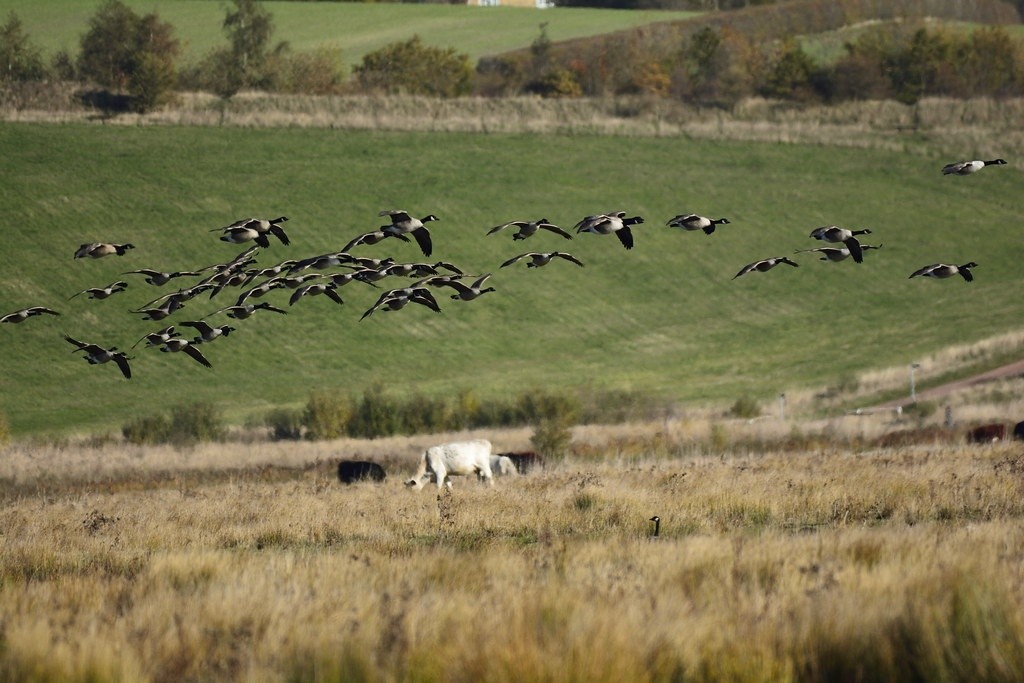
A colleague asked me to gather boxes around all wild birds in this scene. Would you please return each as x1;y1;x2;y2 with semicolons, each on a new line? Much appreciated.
648;515;661;537
730;254;800;280
941;158;1008;176
908;261;979;282
792;225;883;265
0;208;732;382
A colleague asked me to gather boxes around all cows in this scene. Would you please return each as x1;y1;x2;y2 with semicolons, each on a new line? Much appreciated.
402;437;547;492
337;461;388;485
966;420;1024;443
